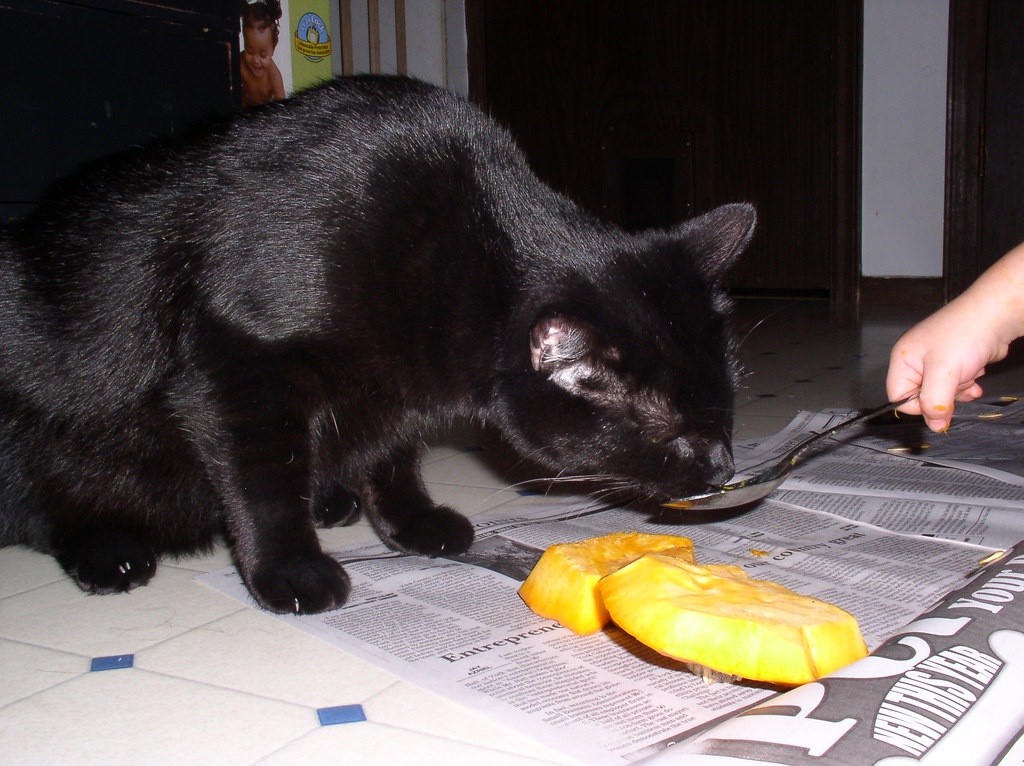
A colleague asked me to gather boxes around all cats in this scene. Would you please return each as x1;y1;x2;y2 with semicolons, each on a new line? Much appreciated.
0;70;758;616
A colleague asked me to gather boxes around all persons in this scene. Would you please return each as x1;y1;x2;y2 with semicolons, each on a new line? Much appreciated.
238;0;285;104
887;241;1024;433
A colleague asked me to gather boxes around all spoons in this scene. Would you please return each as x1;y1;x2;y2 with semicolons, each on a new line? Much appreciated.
658;384;923;511
